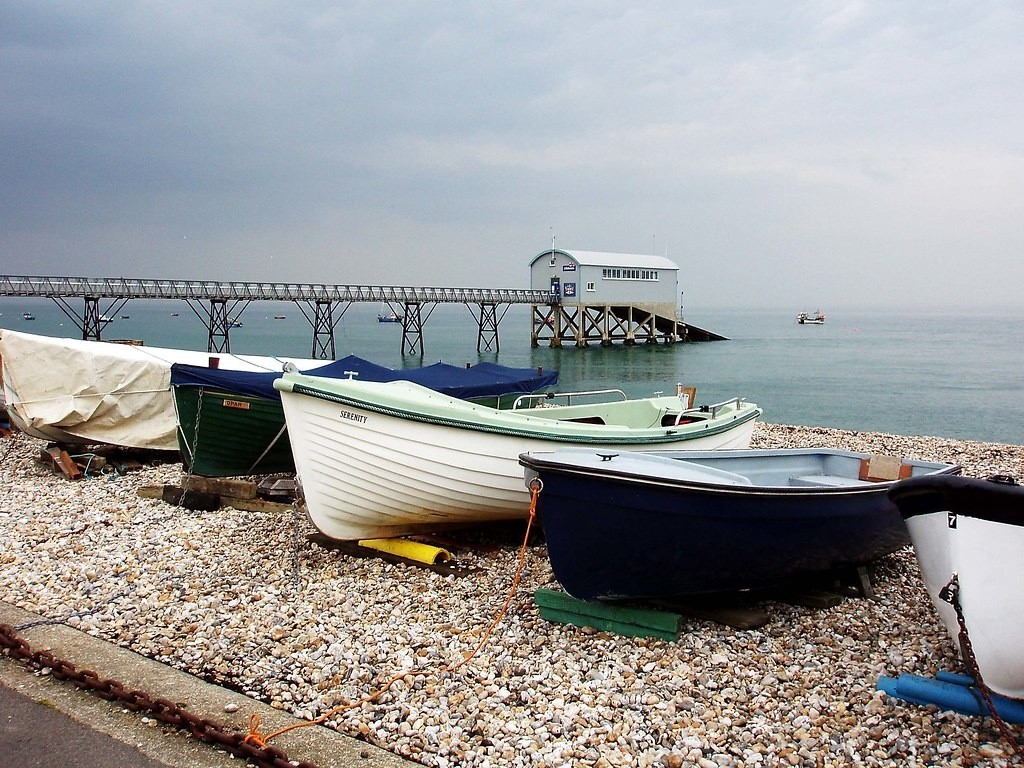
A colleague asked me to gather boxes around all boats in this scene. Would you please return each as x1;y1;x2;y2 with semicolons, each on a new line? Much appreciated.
166;353;562;481
517;444;963;610
884;471;1024;728
0;329;340;445
377;313;404;323
796;312;826;325
271;361;764;544
225;321;244;329
